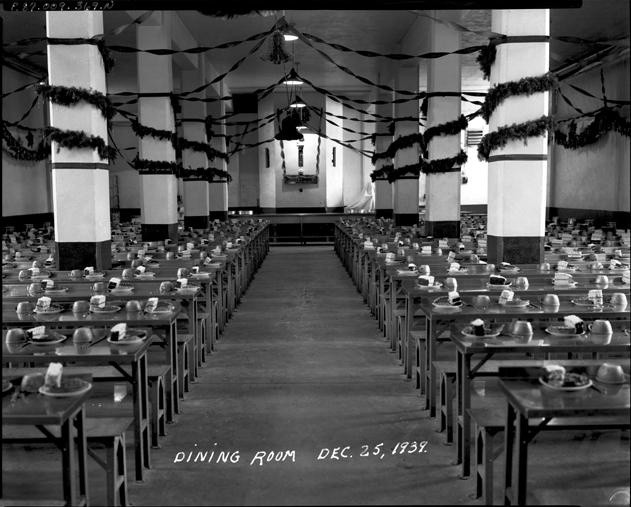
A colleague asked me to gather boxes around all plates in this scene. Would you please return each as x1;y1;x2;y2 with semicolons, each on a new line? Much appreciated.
545;325;586;337
39;381;92;394
462;327;502;338
538;372;593;391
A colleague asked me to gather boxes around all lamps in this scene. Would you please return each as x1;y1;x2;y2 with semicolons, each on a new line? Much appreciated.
261;10;309;141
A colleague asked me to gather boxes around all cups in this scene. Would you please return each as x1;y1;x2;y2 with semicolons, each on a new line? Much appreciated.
3;217;267;346
597;361;627;384
588;320;615;335
512;320;534;337
20;371;44;393
342;212;630;309
